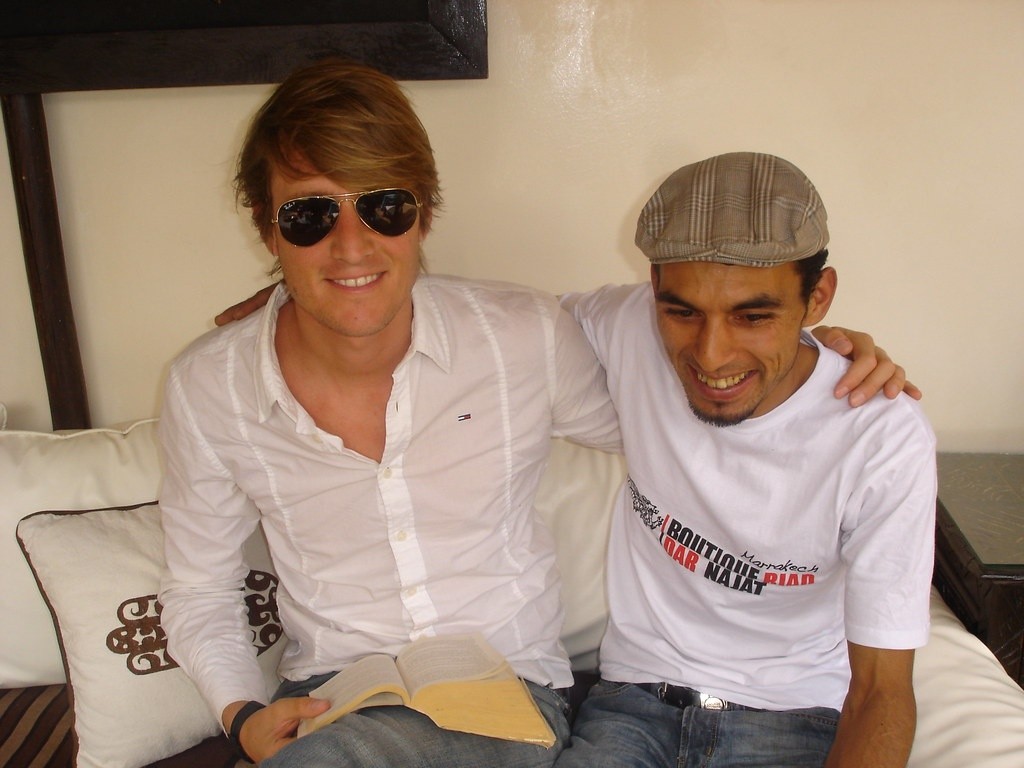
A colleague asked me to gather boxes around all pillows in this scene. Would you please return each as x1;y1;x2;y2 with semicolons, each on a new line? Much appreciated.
16;498;293;768
0;418;159;688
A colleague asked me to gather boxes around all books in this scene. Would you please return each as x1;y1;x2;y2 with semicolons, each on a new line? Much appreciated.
295;632;557;749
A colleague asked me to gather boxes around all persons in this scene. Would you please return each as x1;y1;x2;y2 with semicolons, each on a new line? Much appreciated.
159;60;924;768
211;152;937;768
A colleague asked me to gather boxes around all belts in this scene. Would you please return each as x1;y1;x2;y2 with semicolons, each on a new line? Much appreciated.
639;682;769;714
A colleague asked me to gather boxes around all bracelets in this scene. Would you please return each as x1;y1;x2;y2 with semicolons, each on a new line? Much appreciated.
230;700;265;764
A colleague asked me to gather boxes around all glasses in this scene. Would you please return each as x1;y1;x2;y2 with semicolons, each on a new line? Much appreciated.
271;187;424;247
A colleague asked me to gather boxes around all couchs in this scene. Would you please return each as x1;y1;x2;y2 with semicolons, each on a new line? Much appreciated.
0;592;1024;768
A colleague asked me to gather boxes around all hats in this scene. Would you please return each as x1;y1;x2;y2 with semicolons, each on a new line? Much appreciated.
634;151;830;268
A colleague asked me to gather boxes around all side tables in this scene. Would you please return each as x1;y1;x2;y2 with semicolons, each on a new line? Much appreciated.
937;452;1024;687
